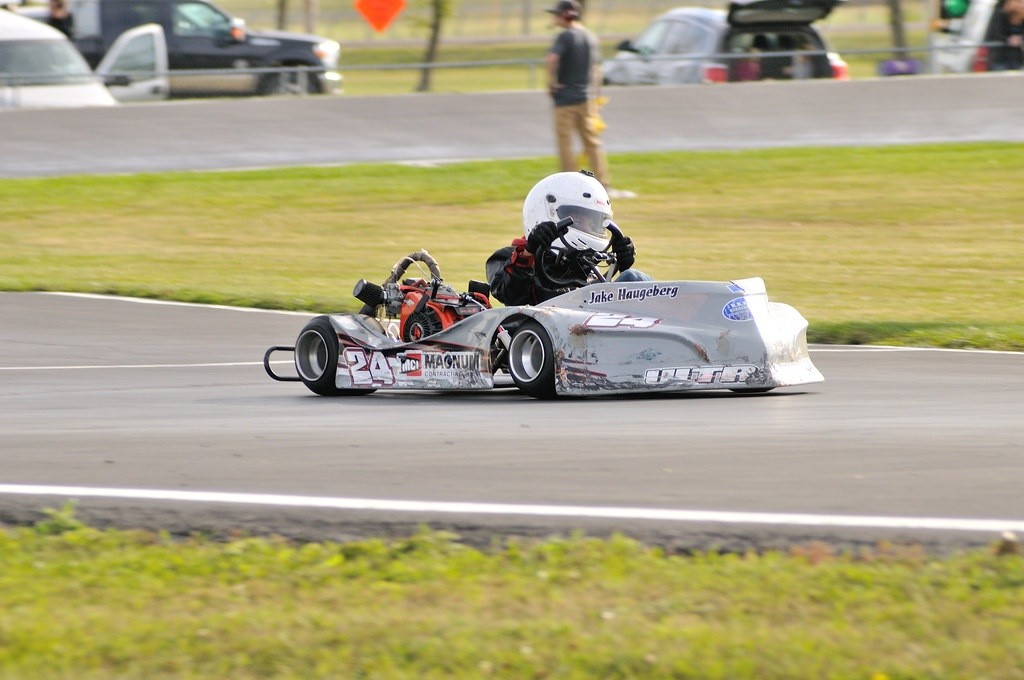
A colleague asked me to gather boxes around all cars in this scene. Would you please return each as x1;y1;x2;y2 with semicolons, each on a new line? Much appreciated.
930;0;1023;78
599;1;852;86
0;3;169;110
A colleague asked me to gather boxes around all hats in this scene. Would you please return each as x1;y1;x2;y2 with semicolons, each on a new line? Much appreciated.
546;1;582;19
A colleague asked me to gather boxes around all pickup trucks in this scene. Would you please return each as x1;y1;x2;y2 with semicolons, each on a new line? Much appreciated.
24;0;343;100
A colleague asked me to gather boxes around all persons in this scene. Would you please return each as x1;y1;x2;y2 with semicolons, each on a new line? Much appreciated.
783;44;819;79
544;1;638;198
485;171;653;306
47;0;73;38
930;0;971;74
732;47;773;82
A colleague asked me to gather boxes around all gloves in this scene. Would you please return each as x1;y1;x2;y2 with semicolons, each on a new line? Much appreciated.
525;221;569;256
611;237;635;272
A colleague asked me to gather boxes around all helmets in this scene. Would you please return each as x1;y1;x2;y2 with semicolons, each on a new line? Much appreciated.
522;169;613;255
